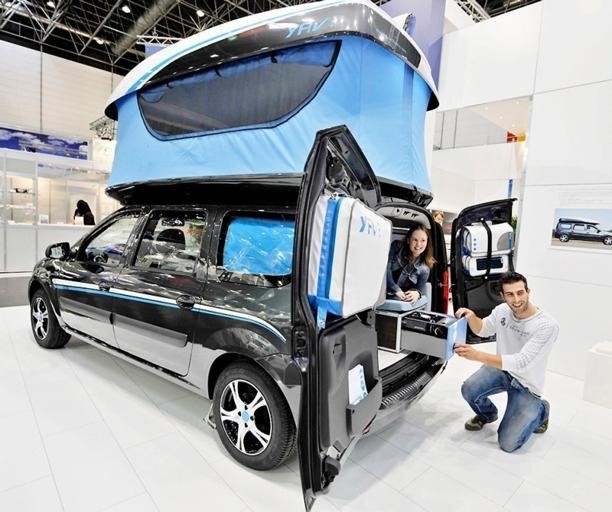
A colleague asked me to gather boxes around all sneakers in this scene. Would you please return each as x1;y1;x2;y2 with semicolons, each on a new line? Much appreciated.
465;414;497;430
534;400;549;433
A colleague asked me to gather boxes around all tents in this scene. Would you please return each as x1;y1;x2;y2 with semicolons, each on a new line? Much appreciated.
103;0;440;209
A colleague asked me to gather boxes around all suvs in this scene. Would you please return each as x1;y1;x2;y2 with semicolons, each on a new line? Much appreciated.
21;123;521;512
554;218;612;246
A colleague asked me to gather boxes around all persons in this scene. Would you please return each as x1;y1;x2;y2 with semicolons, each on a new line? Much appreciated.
73;200;95;225
387;224;438;305
453;272;559;453
434;211;445;224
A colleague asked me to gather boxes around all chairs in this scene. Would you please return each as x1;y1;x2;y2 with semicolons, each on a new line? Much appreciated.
158;230;185;255
